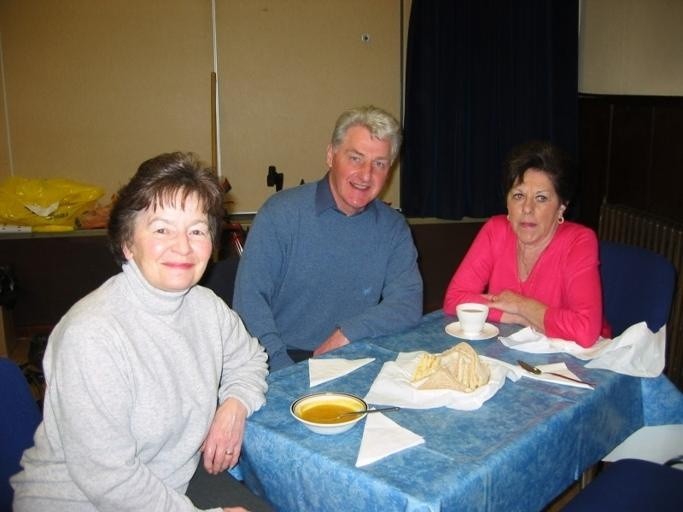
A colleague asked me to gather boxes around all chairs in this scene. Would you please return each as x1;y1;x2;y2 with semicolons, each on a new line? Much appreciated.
0;353;45;511
591;245;675;360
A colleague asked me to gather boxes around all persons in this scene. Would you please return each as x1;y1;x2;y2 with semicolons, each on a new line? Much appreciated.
443;146;612;348
232;105;424;373
9;152;269;512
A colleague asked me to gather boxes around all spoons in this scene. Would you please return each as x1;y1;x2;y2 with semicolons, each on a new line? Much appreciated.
516;358;598;390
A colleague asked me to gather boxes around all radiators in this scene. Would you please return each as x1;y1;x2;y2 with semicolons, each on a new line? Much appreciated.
589;198;681;387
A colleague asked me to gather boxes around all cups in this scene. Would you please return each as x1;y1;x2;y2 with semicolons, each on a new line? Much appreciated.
456;304;489;332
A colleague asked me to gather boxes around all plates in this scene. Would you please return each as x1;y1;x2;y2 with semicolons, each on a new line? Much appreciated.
444;321;500;343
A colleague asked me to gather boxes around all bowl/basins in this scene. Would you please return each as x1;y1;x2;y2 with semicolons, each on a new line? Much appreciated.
289;393;367;437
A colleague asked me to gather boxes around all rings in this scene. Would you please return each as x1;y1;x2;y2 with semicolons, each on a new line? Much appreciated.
492;296;497;301
226;451;233;455
